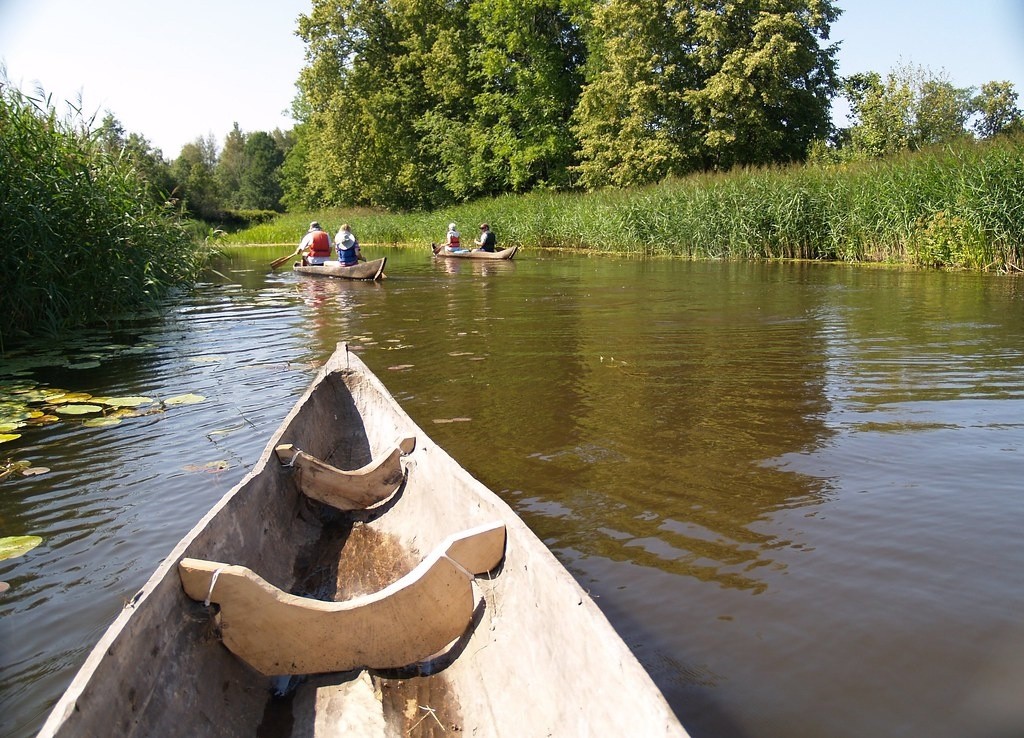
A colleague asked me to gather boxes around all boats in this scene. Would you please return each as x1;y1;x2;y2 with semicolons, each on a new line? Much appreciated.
293;257;387;283
430;240;517;261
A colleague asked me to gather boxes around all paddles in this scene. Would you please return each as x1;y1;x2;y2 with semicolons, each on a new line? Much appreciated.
432;240;448;256
269;246;308;271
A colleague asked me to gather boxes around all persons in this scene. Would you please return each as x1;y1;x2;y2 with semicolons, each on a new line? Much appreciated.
333;224;367;266
440;223;461;254
471;223;497;253
296;222;332;266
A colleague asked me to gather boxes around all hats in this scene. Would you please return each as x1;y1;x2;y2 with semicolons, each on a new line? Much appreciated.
335;230;355;250
308;222;322;233
449;223;456;231
479;224;489;230
